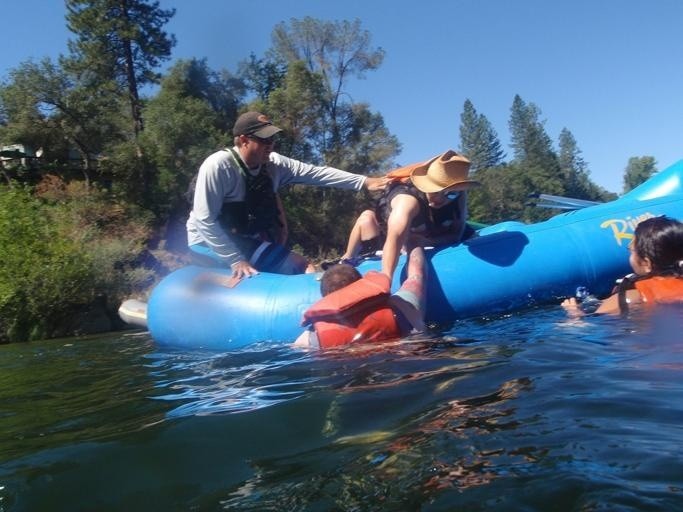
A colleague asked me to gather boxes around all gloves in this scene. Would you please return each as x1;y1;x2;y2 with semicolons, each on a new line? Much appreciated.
140;155;683;352
115;295;148;329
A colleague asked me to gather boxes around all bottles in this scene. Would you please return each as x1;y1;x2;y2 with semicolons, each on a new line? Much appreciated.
411;150;481;193
233;111;282;139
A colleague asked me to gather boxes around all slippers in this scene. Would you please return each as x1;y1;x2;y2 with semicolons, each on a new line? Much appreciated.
229;149;283;242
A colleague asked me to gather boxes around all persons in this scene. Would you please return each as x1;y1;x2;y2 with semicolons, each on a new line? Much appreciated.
183;111;394;277
560;215;683;315
293;242;425;347
319;149;483;271
